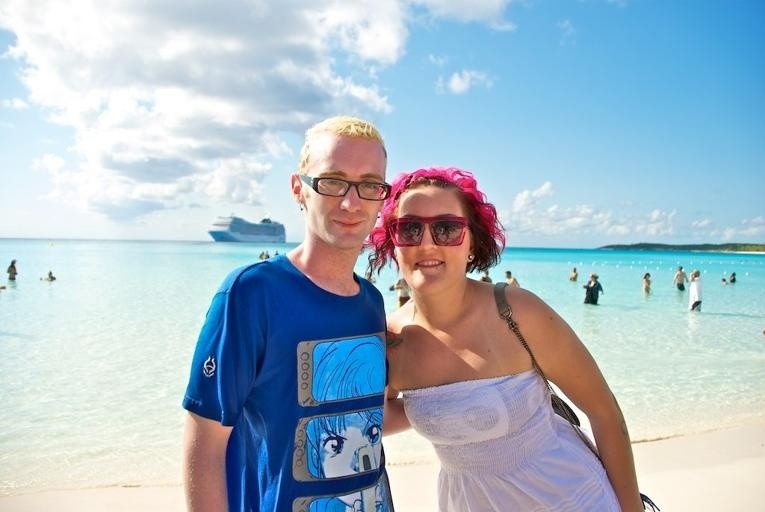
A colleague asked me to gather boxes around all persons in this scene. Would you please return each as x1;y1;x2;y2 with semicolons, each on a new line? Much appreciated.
6;259;18;280
361;167;658;511
389;276;411;306
39;270;56;282
183;117;411;512
480;266;736;311
259;249;279;260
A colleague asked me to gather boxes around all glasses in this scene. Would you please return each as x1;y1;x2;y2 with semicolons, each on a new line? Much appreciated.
386;216;468;246
298;171;392;200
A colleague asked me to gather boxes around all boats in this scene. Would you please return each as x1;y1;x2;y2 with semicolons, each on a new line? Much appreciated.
208;216;285;242
